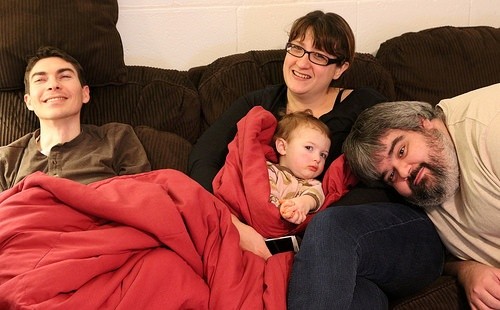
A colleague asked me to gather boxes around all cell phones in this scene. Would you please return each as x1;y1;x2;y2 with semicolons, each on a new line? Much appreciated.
265;235;299;255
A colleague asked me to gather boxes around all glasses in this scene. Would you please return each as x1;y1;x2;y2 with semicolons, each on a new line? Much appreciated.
284;43;345;66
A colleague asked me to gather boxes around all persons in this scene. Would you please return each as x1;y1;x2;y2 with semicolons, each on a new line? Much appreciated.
260;110;332;224
342;77;500;310
0;45;151;200
191;10;444;309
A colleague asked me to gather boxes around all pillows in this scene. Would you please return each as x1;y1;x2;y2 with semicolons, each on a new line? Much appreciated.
0;0;127;93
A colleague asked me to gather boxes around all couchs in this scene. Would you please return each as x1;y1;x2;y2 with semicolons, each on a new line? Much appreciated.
0;23;499;310
